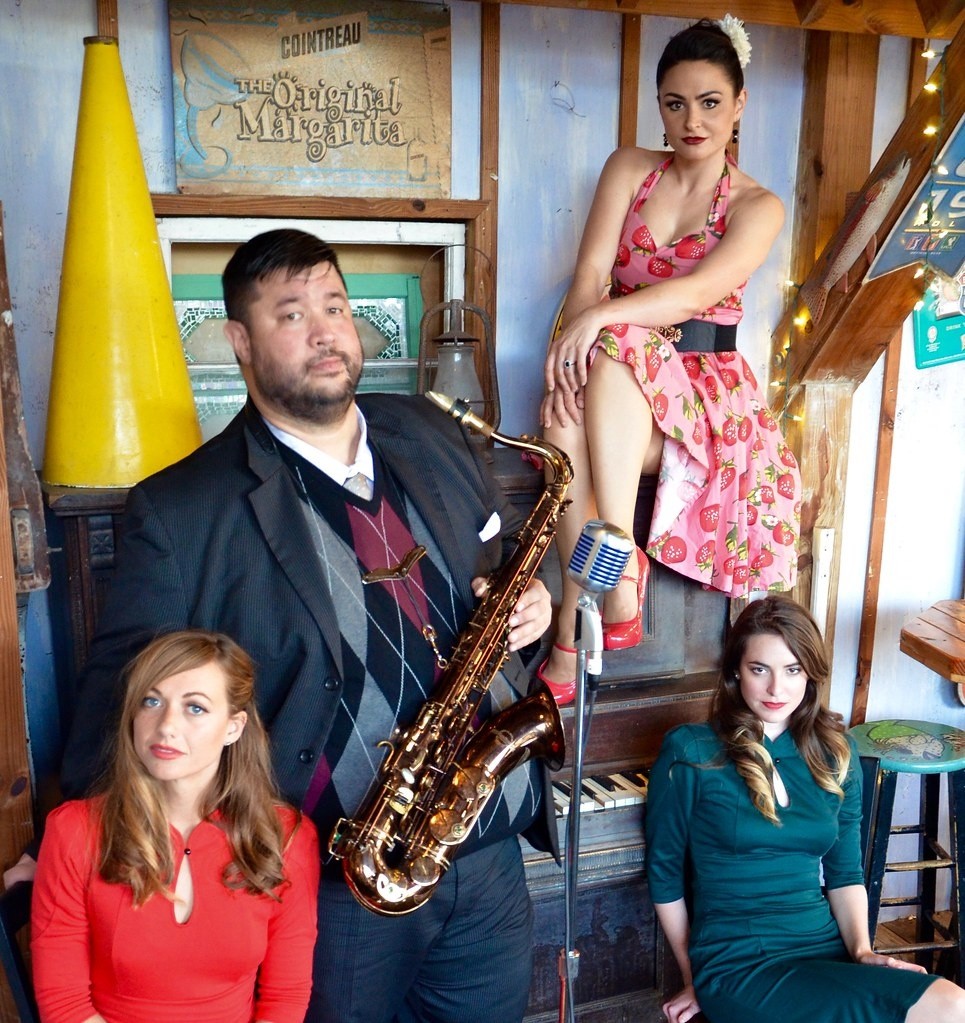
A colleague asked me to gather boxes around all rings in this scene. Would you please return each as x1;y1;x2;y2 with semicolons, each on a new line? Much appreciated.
564;360;576;368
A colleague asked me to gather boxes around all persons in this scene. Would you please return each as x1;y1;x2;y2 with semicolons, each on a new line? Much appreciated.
30;630;320;1023
540;16;801;704
3;230;564;1023
638;595;964;1023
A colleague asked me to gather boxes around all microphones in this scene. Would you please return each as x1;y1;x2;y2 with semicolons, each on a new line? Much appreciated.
567;518;637;690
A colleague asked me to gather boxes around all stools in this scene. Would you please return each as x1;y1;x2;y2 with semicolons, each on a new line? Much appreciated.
847;720;965;957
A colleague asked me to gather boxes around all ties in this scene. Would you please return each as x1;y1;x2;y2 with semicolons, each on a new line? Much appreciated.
345;472;375;501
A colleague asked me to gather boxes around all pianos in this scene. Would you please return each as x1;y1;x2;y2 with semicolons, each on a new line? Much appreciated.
32;444;753;1023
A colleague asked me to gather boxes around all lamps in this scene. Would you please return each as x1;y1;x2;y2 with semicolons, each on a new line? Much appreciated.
416;244;501;465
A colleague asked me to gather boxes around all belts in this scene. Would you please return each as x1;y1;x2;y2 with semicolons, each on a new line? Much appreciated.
669;318;737;351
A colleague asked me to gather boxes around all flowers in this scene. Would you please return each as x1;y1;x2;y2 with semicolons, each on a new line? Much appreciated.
716;14;751;68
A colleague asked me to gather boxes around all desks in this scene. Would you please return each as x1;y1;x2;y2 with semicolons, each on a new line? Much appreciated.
899;601;965;682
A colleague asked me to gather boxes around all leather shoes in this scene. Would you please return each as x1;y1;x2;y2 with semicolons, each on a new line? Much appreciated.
536;642;592;706
600;545;650;651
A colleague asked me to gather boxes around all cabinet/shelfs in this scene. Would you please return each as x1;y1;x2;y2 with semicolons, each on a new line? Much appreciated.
37;470;129;681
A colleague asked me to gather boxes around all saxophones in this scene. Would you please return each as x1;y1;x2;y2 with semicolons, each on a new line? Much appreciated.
325;389;576;918
